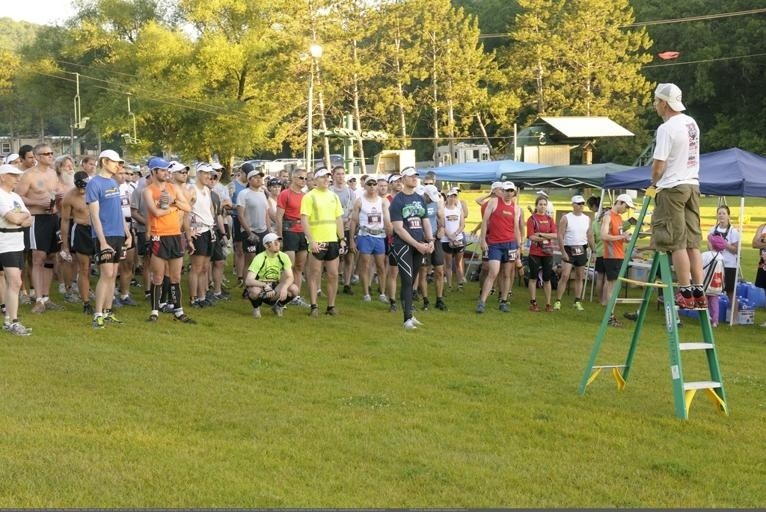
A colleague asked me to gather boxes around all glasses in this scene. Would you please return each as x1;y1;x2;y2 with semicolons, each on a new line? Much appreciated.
180;171;189;176
575;202;587;207
9;159;23;165
505;189;515;194
424;182;435;186
124;172;135;177
37;152;55;157
266;238;282;245
27;155;36;160
282;174;290;179
365;182;379;187
214;169;224;175
208;177;217;182
293;175;309;182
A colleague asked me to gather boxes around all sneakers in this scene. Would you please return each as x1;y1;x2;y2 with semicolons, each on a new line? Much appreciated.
325;307;339;316
662;321;682;329
149;310;159;322
130;280;143;288
198;299;208;308
45;299;66;313
158;300;167;312
88;289;96;300
435;301;448;313
694;296;709;309
545;304;554;313
712;323;718;329
253;307;261;320
0;305;8;315
189;301;201;311
403;318;419;331
105;312;124;326
623;310;638;322
72;282;79;292
119;295;136;307
390;303;398;314
379;295;389;305
343;284;355;296
174;312;196;325
411;289;420;302
607;315;624;328
215;293;228;301
499;302;510;313
553;301;561;311
657;293;696;310
63;291;82;305
310;308;319;321
475;301;487;314
222;276;232;283
423;303;430;312
31;300;47;315
92;312;107;330
161;304;175;314
274;304;285;318
58;281;67;295
364;293;372;303
2;320;34;337
221;290;233;300
292;297;311;309
573;301;585;312
83;302;91;316
221;282;230;289
317;289;327;299
373;275;379;285
529;302;540;313
112;298;124;308
350;273;360;284
115;287;122;292
19;293;31;307
31;292;39;302
411;316;424;327
205;291;220;305
144;293;152;301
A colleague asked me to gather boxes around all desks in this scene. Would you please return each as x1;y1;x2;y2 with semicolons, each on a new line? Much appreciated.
625;261;664;312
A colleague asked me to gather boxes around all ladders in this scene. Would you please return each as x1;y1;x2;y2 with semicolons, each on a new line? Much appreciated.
580;185;731;419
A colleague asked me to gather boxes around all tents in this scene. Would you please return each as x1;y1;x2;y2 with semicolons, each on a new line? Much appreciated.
415;159;551;183
604;146;766;328
501;161;650;301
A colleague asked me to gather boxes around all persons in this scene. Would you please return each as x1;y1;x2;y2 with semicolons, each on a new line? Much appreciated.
526;196;557;313
141;157;192;324
236;170;270;299
131;165;142;183
450;186;468;219
276;168;308;296
86;149;132;330
3;153;23;168
552;195;596;313
427;172;437;184
475;181;521;313
267;177;283;236
54;154;96;303
82;156;96;177
208;168;228;309
389;166;424;330
442;190;466;293
375;178;389;293
528;190;554;218
210;162;233;304
304;172;315;190
15;145;37;307
300;166;345;318
422;175;434;186
355;175;369;196
476;182;502;303
184;161;214;310
230;168;240;178
61;170;95;316
649;83;708;310
419;186;449;312
130;174;170;302
349;177;393;305
708;205;738;300
586;195;601;218
751;223;766;290
591;206;611;307
125;166;136;191
600;194;636;328
0;163;33;337
245;232;298;318
113;164;137;306
17;144;66;315
497;207;525;307
166;163;196;313
701;234;728;329
278;170;290;189
329;166;355;296
227;163;256;287
386;172;418;313
347;175;360;197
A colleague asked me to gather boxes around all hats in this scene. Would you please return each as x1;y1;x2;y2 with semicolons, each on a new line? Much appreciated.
401;167;421;178
500;181;518;192
211;161;226;170
364;176;378;184
207;164;215;173
196;162;215;173
571;195;586;205
376;175;389;183
488;182;502;197
415;185;425;196
707;233;727;252
536;190;550;198
268;177;283;187
73;171;90;189
447;191;459;197
149;158;172;174
99;149;126;166
169;164;191;174
450;187;462;193
616;193;636;209
247;170;265;183
423;184;441;204
346;175;358;186
388;174;402;185
6;153;19;164
169;160;181;165
654;82;687;112
313;168;333;179
2;156;7;164
0;163;25;176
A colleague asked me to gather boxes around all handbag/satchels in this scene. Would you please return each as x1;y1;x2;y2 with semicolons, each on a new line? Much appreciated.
359;225;385;236
0;227;31;252
566;244;586;256
448;233;463;248
282;220;302;232
531;212;554;250
